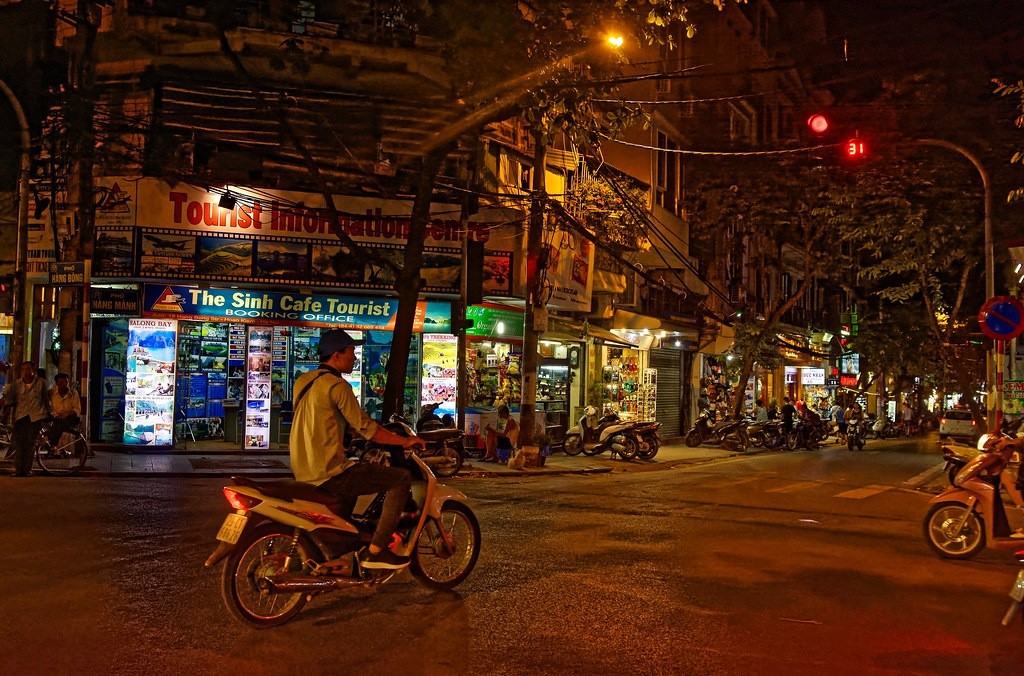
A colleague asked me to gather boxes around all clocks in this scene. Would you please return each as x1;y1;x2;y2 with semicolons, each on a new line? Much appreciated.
569;346;579;369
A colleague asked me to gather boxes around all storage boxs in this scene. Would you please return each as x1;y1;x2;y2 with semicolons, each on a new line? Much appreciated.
515;446;539;466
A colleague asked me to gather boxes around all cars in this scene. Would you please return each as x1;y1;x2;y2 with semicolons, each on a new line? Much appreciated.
939;407;987;441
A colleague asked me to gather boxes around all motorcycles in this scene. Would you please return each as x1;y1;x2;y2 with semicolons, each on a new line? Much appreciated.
562;404;664;462
922;417;1024;625
685;404;830;451
204;423;482;630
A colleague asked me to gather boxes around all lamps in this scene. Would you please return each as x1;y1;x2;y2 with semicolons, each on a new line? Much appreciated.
217;182;237;210
490;341;496;348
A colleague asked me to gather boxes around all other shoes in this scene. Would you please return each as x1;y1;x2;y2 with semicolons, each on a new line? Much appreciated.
15;468;32;477
478;455;498;463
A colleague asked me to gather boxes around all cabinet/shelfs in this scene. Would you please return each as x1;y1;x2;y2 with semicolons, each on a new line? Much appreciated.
535;364;567;404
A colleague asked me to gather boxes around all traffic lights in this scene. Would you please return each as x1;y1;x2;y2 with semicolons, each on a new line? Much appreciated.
805;114;833;137
0;283;13;294
843;139;868;160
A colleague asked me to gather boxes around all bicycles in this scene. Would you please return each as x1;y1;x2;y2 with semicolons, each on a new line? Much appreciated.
0;418;89;477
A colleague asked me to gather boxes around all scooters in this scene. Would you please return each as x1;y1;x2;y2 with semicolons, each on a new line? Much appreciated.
848;418;865;451
862;415;893;440
359;401;464;479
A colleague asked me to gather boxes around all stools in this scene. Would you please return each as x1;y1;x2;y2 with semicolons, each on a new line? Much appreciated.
540;442;552;457
497;447;515;465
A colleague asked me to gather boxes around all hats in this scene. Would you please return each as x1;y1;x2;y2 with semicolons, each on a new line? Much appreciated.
316;330;365;353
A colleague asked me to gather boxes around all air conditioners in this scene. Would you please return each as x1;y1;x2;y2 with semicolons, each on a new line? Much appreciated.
577;295;614;318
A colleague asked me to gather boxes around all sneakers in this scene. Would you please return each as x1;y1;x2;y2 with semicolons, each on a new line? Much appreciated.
361;547;411;570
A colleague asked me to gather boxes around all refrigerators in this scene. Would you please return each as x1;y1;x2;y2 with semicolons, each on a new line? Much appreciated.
464;406;546;442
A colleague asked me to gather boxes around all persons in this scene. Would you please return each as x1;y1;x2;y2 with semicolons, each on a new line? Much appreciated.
829;400;866;445
3;362;48;478
781;396;823;447
844;357;857;374
289;329;426;570
901;401;916;437
42;373;81;452
478;405;519;463
750;399;780;421
996;435;1024;540
271;378;285;406
